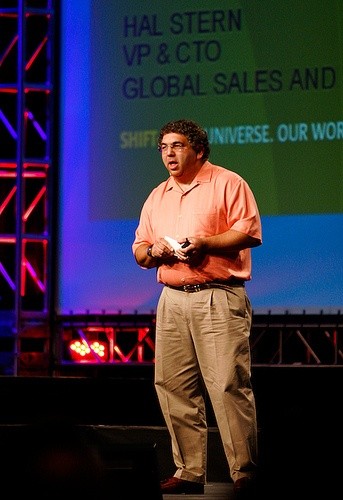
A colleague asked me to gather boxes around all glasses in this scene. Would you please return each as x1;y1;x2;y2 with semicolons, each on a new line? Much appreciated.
160;144;191;153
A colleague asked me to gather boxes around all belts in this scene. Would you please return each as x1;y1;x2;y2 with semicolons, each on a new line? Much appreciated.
164;281;242;293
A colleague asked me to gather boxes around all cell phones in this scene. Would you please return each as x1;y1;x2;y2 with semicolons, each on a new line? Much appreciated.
182;238;190;254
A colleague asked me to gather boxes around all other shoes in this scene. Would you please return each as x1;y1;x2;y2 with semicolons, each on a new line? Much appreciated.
234;477;250;494
160;477;204;495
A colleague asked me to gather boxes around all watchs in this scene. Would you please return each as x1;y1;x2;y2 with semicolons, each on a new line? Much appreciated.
146;243;155;258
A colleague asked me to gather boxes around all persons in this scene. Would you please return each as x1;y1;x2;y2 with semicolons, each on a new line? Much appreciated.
131;117;264;494
